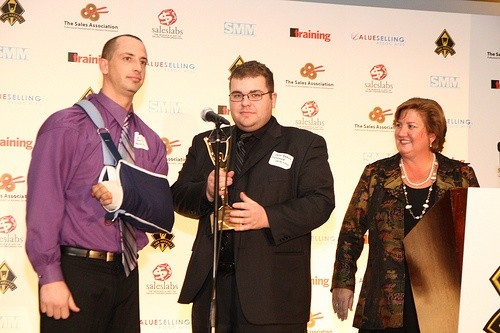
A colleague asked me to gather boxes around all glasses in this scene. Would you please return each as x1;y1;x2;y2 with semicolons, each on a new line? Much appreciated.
228;91;273;102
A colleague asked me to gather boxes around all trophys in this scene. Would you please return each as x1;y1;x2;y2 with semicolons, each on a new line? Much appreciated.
203;126;242;233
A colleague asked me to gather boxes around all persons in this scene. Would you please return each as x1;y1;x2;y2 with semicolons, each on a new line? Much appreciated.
330;98;480;333
24;35;168;333
170;59;336;333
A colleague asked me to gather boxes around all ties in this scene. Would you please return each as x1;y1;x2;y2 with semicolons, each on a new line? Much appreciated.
220;131;255;252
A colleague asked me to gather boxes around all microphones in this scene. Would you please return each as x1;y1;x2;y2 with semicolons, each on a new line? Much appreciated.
200;107;230;126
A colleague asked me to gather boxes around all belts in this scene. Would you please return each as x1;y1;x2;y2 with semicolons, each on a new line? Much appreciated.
217;261;236;273
60;244;139;263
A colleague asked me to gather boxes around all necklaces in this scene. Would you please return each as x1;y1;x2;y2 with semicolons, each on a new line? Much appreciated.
401;153;436;186
399;160;438;219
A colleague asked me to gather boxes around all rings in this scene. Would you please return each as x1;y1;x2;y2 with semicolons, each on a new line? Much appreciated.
334;304;339;306
